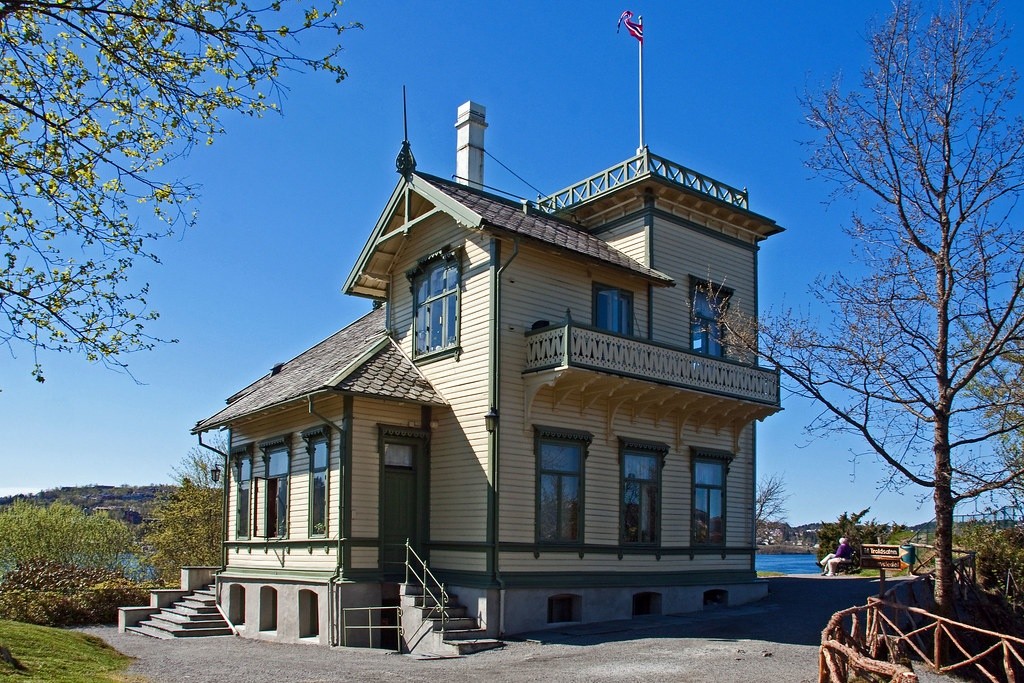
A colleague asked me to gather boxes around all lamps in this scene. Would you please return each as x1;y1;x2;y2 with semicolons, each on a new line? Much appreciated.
484;403;500;435
211;464;224;483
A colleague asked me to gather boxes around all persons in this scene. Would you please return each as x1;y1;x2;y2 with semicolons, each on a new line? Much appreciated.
815;537;852;576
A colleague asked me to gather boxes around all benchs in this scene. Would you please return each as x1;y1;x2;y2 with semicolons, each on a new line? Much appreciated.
835;550;856;574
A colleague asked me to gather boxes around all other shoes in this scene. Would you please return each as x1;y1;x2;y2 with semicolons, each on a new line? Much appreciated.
816;561;823;569
826;573;834;577
821;572;828;575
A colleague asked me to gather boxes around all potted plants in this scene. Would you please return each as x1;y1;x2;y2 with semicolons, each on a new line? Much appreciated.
314;523;325;534
274;525;284;537
